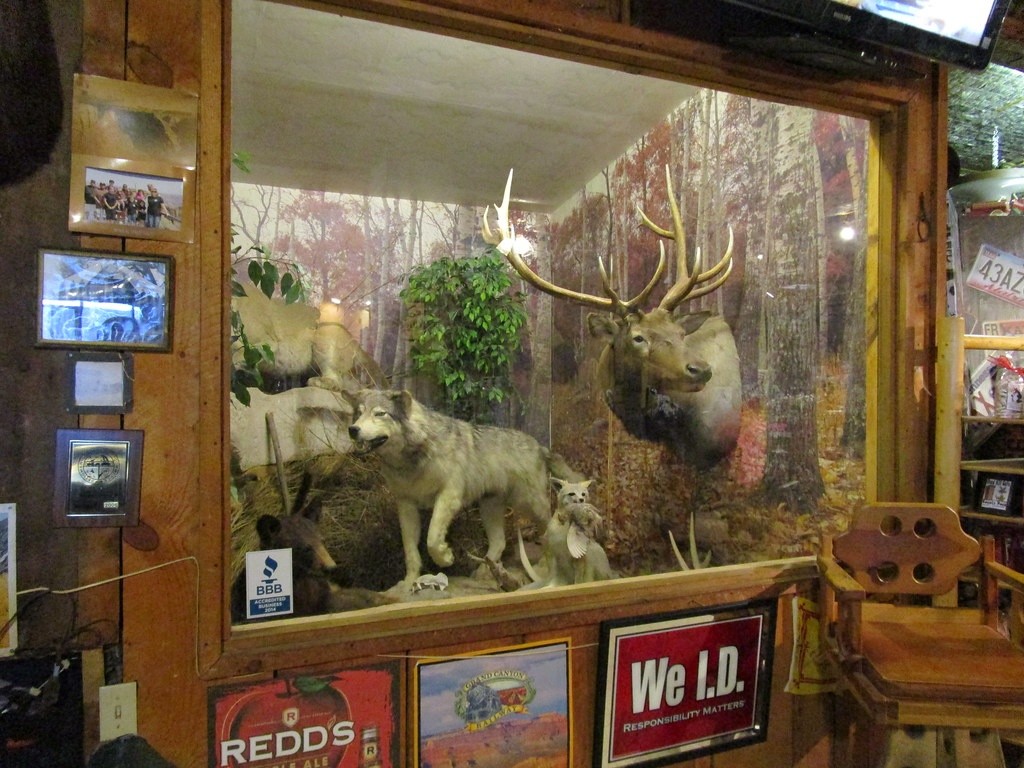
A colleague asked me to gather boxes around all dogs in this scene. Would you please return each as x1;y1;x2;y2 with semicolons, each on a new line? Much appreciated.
340;387;587;591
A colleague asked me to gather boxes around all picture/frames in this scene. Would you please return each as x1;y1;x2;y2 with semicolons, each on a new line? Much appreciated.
590;595;781;767
34;246;177;354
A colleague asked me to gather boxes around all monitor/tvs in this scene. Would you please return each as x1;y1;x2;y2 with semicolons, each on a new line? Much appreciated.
723;0;1012;73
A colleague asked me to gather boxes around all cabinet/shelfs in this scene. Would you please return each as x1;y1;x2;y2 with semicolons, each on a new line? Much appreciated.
932;314;1022;609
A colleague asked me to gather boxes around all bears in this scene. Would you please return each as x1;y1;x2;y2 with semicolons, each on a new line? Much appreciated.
230;495;338;629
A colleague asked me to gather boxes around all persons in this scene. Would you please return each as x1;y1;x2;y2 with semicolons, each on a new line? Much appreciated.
84;180;170;227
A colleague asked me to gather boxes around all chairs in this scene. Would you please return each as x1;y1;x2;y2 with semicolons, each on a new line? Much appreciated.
814;500;1024;767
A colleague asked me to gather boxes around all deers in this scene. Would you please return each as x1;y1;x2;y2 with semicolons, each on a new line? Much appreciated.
481;161;744;468
